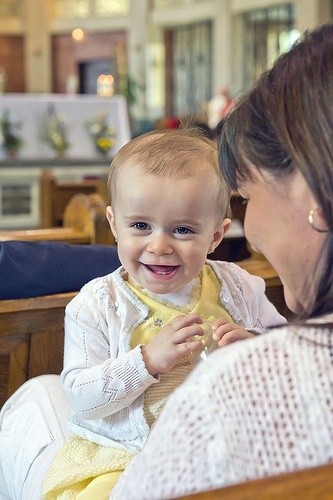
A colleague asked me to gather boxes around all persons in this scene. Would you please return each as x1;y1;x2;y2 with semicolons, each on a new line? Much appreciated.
0;22;333;500
60;129;288;452
206;85;232;130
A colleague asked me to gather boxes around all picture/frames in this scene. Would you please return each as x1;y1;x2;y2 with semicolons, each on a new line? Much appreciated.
0;94;130;167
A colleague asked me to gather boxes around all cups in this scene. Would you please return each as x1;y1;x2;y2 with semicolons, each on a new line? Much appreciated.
186;320;223;368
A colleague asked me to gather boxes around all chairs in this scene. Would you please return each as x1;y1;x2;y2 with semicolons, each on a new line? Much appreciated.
1;170;332;500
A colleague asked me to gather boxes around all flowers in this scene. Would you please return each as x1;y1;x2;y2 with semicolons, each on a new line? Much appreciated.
37;111;69;153
0;110;25;153
86;109;116;152
